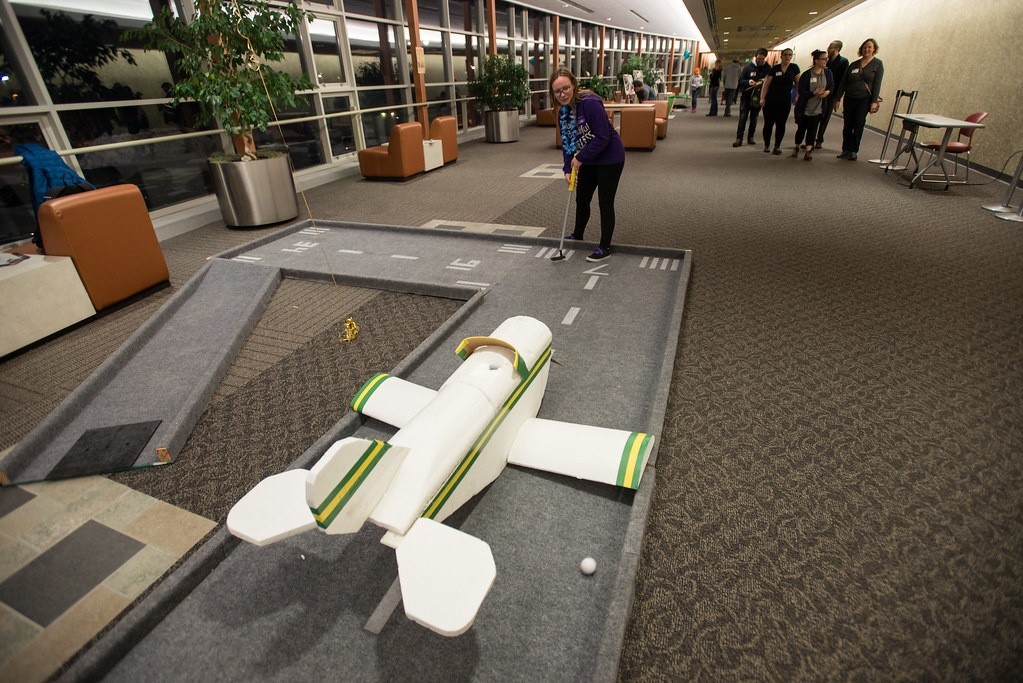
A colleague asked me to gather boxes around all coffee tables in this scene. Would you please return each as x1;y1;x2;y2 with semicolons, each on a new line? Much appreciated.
381;140;444;171
0;252;97;359
603;103;655;136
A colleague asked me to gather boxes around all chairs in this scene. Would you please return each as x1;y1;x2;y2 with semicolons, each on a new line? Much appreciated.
913;113;989;183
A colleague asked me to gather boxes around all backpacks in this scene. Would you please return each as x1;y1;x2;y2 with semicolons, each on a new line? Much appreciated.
28;171;97;251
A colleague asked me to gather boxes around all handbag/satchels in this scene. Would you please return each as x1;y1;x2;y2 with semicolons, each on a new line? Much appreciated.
869;96;885;114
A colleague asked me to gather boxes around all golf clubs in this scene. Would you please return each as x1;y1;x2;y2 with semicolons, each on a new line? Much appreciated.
551;153;578;261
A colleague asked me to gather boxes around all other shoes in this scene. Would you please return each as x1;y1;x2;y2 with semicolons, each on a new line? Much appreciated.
791;147;799;158
732;139;743;147
804;152;812;160
691;108;697;113
813;144;822;149
723;113;732;117
763;147;770;152
836;151;848;158
848;152;858;159
772;149;781;155
800;144;807;149
747;138;755;144
705;113;717;117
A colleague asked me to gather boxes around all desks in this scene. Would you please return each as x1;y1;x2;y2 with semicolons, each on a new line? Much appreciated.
894;113;985;190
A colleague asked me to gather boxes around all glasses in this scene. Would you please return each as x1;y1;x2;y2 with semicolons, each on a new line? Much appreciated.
553;83;573;98
817;58;830;61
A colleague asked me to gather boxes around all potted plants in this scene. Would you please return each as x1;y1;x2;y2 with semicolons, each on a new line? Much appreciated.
120;1;321;227
466;53;533;142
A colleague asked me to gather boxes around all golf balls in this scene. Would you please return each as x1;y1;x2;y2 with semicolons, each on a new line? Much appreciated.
580;558;597;574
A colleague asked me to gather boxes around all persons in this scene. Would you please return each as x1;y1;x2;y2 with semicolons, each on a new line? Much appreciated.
705;60;722;116
5;91;38;150
833;39;884;159
733;48;771;147
791;49;835;161
760;48;800;155
549;68;625;262
800;41;849;150
690;68;704;113
161;83;194;153
95;82;156;160
721;59;743;117
632;81;656;104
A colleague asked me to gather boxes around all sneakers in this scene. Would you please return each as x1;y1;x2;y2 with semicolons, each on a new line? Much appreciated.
586;246;612;263
563;235;584;242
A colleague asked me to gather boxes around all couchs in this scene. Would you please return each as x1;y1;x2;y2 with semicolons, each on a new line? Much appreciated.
536;100;669;152
4;184;169;358
358;115;458;179
